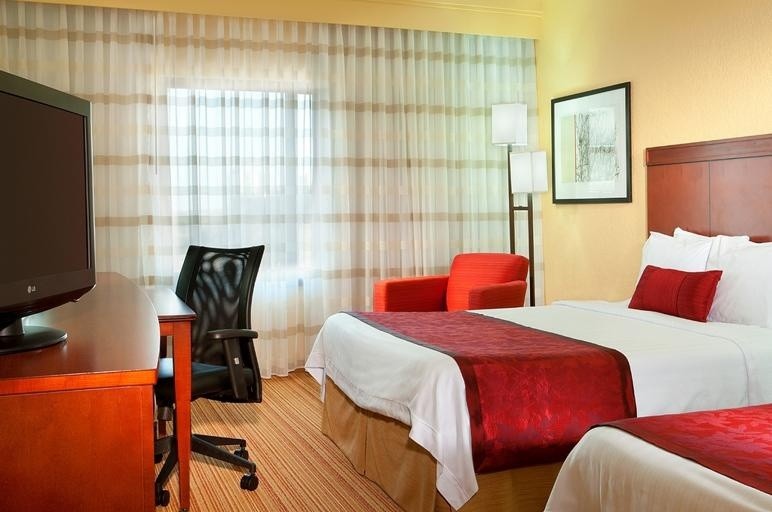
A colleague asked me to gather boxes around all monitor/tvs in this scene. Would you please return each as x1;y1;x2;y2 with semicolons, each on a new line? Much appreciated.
0;69;96;355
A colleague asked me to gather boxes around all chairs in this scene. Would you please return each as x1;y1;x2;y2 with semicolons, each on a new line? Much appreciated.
148;244;270;506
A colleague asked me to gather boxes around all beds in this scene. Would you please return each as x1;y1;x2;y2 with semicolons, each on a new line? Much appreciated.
298;131;772;511
539;401;772;511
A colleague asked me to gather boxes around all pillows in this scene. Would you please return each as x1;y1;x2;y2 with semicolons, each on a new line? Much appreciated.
634;231;714;293
702;232;771;330
673;224;749;247
622;264;722;324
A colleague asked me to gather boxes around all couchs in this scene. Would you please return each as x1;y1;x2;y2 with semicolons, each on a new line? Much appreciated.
371;249;530;313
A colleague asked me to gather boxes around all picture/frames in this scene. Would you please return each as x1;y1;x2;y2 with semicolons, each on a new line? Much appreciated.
545;81;633;205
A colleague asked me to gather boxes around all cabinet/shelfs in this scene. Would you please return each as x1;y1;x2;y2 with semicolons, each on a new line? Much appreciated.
0;270;164;512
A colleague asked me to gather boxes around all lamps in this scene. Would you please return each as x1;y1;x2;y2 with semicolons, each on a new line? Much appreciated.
507;148;550;306
488;101;528;254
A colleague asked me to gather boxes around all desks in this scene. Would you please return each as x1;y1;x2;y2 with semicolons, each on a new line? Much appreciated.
134;283;199;511
